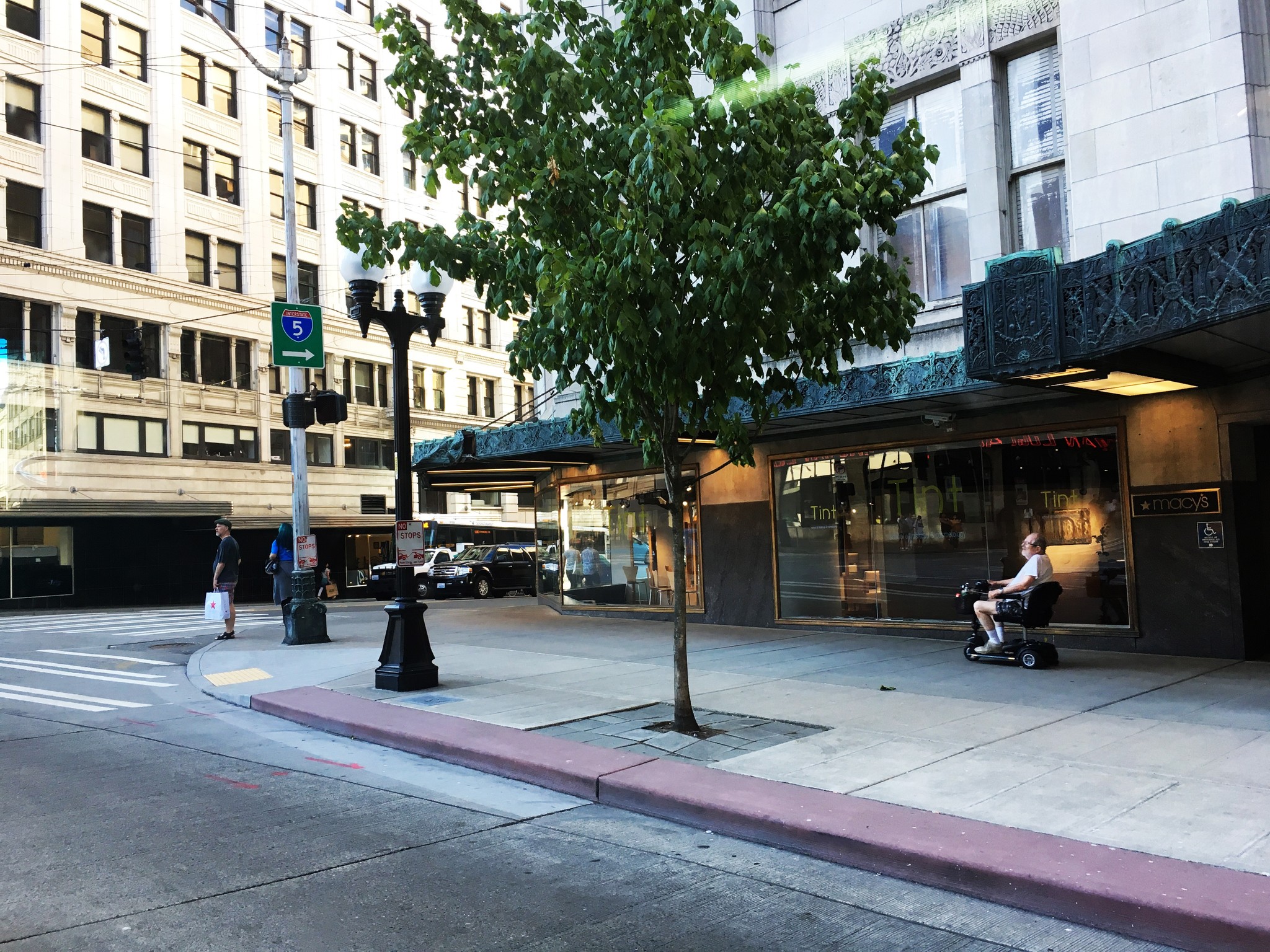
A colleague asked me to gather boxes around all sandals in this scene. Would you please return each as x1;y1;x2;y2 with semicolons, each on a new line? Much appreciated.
214;630;235;640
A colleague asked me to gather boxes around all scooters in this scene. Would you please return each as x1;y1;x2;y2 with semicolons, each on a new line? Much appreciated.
954;577;1063;670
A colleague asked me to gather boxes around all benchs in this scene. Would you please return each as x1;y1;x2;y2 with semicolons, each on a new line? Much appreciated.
845;569;880;611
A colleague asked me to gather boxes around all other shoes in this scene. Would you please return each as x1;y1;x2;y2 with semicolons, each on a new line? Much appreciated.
317;597;321;600
332;596;336;600
974;640;1003;654
326;581;338;597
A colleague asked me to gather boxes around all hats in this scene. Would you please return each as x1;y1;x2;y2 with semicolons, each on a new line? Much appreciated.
214;518;232;529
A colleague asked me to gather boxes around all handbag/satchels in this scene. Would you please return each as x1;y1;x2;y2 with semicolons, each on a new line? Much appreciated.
205;587;230;620
265;544;281;574
572;560;583;576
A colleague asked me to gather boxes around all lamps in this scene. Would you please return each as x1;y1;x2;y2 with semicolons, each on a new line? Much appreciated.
606;500;612;507
573;500;578;505
619;499;625;509
588;499;595;508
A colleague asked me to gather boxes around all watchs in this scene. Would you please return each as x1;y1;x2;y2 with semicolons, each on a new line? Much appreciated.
999;587;1004;594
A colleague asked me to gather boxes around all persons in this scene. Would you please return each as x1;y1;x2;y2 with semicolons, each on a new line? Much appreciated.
897;513;962;551
317;563;336;600
974;533;1053;654
213;519;241;640
563;538;601;588
270;523;294;625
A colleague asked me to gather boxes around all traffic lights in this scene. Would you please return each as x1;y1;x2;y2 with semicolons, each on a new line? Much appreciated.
315;392;348;425
123;337;147;381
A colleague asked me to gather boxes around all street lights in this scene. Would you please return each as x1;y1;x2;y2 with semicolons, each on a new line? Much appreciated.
339;233;457;693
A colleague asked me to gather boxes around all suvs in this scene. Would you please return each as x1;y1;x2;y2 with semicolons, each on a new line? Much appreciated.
368;541;474;601
426;540;558;600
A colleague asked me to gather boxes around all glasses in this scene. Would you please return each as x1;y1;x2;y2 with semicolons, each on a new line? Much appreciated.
1022;540;1042;549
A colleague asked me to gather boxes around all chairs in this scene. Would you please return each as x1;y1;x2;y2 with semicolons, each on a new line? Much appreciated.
666;571;699;607
621;565;649;605
652;569;672;606
646;567;671;605
